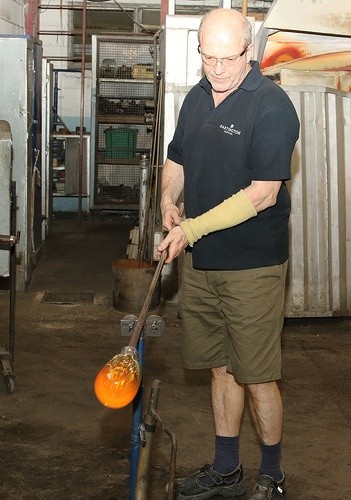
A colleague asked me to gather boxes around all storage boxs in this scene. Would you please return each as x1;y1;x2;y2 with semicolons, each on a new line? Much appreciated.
102;127;139;158
132;63;155;80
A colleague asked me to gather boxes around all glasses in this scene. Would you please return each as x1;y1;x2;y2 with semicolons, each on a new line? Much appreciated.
196;45;248;66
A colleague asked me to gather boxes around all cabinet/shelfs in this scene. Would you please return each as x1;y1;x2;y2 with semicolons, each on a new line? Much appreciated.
88;34;159;223
49;131;88;196
1;33;47;293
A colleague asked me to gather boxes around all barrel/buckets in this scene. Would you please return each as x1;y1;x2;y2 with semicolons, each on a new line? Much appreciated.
111;258;160;314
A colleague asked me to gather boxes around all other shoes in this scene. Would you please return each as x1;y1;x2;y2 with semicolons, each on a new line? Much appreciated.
248;471;286;500
165;463;245;500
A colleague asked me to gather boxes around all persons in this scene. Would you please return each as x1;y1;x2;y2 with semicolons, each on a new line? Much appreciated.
155;8;301;500
